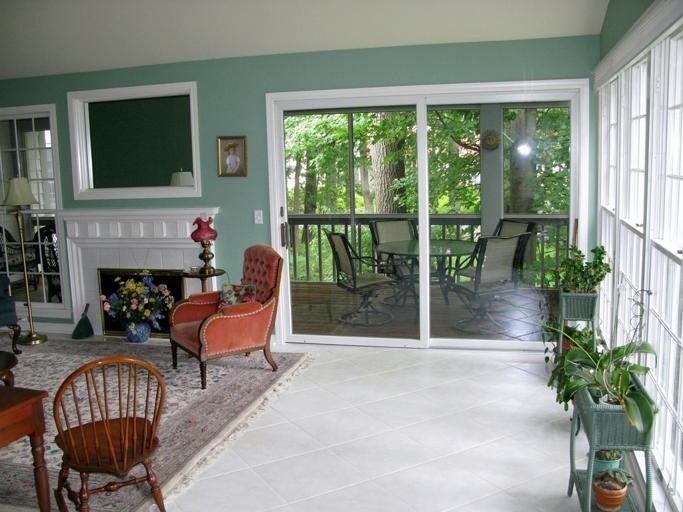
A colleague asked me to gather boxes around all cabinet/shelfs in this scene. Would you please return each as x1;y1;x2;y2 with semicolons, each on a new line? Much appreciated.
568;359;655;512
559;283;598;359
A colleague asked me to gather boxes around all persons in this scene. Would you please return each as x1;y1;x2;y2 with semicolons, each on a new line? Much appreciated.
224;142;241;176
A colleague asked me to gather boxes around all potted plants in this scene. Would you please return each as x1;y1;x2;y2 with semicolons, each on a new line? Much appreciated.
563;324;579;351
599;344;658;434
587;449;623;469
593;469;632;510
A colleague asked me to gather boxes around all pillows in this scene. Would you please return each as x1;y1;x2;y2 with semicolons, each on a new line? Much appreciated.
218;284;256;312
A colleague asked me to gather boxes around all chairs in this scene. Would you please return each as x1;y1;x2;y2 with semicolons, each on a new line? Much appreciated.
169;245;283;389
0;225;40;291
323;217;536;335
0;272;22;355
54;356;165;512
34;226;61;302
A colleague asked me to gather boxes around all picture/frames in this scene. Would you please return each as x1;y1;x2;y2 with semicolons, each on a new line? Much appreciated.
217;136;248;177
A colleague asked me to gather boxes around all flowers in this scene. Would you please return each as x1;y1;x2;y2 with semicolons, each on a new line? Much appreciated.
99;270;174;335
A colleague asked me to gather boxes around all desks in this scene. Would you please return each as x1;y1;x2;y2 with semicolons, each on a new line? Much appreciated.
0;385;51;511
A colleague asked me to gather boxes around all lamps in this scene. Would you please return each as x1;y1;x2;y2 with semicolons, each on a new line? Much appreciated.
1;177;48;346
170;167;194;186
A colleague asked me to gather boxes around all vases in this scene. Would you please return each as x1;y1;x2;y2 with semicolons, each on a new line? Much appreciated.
125;320;151;343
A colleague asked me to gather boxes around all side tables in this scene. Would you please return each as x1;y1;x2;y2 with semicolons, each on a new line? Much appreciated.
181;269;225;293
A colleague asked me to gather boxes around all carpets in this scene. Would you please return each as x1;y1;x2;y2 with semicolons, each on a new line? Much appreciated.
0;332;312;512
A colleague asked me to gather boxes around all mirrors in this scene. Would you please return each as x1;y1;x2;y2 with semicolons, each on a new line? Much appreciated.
66;80;202;201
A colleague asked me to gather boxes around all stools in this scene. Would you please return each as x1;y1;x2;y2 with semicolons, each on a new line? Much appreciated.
0;351;18;387
